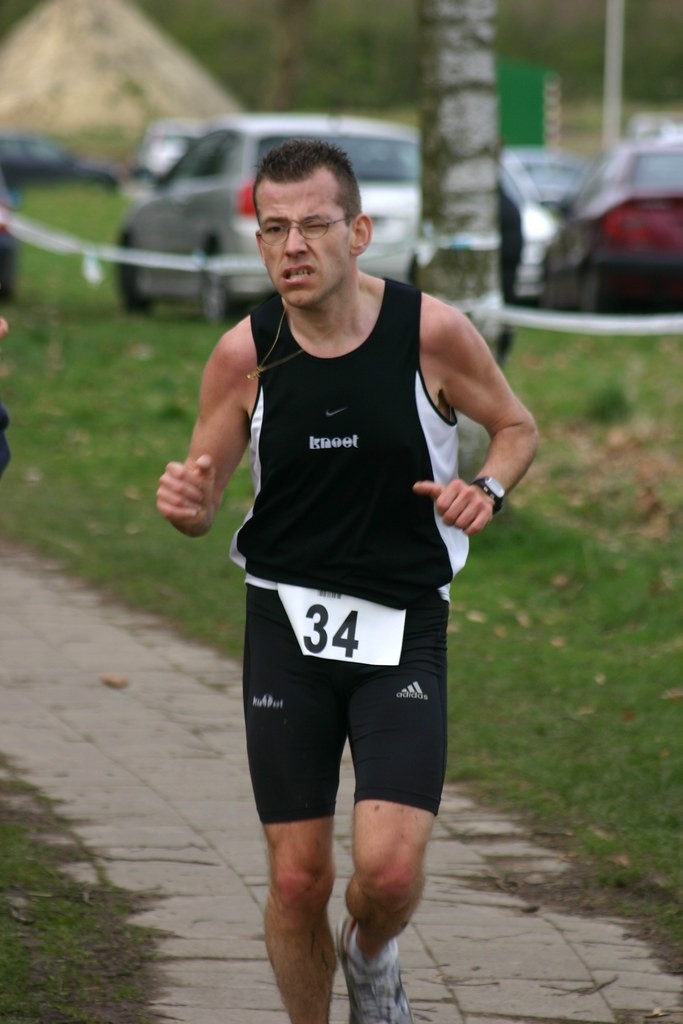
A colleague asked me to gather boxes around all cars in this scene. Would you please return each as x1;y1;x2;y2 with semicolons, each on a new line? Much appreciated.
115;112;422;329
540;126;683;316
0;118;209;321
501;141;596;295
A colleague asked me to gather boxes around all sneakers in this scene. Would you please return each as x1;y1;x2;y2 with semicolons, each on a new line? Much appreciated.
336;913;414;1024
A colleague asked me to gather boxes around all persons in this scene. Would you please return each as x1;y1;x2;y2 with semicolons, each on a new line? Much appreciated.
0;228;15;476
157;138;539;1024
498;137;524;364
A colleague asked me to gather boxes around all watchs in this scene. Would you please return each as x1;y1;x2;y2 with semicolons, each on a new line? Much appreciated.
470;475;505;514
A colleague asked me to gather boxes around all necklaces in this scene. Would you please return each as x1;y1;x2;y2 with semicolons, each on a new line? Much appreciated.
246;276;365;381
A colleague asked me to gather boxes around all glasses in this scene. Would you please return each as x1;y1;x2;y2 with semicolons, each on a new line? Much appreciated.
257;216;358;247
